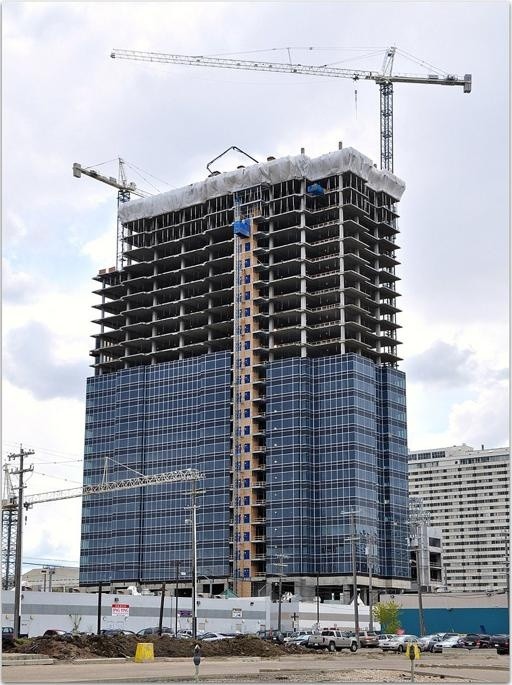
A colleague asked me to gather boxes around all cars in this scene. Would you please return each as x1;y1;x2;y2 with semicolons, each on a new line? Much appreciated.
43;627;237;640
256;628;509;655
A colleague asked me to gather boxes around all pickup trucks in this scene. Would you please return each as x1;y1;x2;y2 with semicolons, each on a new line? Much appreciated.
2;627;28;640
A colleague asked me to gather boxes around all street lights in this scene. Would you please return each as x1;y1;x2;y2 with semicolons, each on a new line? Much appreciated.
184;518;197;639
409;559;425;637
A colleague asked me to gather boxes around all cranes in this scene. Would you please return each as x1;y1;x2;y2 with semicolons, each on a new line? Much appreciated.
1;456;206;590
110;45;473;174
72;156;178;270
